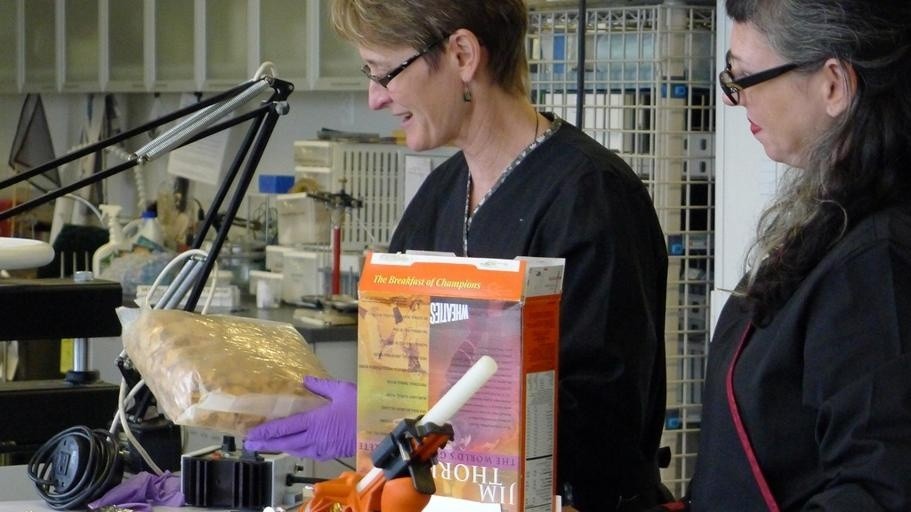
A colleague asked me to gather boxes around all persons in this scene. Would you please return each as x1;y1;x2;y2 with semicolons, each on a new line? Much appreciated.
676;1;910;511
242;1;675;511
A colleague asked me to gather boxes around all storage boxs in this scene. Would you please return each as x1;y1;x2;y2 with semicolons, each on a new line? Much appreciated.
356;248;567;512
264;138;407;309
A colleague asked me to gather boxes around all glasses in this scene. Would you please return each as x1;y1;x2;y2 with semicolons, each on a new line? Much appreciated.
719;50;806;105
360;38;443;88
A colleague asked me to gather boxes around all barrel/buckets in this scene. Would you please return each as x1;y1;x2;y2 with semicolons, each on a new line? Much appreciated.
123;210;166;255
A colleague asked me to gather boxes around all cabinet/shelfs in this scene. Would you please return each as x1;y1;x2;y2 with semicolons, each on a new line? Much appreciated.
524;0;717;500
0;0;369;94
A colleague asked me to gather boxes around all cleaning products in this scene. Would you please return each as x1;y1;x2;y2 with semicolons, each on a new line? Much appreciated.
90;203;132;279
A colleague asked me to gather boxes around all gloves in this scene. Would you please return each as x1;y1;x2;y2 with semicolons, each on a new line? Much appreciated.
87;469;185;512
244;376;357;463
